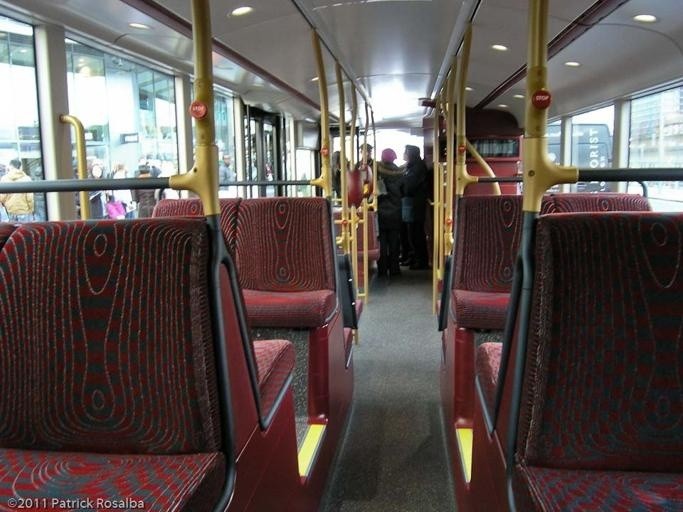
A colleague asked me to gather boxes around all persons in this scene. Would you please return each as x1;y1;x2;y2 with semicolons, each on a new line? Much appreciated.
0;152;166;230
397;145;429;270
332;151;353;207
394;161;412;269
356;143;376;168
372;147;406;282
218;154;235;191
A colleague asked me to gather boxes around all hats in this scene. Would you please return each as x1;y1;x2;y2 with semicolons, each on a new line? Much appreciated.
360;144;372;150
382;149;396;161
407;145;420;158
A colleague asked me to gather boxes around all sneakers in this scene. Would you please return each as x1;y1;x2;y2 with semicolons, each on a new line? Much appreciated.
378;254;429;277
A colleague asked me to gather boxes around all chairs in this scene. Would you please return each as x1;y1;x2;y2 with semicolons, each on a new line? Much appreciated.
0;193;683;512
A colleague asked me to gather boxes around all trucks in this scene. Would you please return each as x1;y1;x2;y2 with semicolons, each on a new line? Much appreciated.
13;116;142;180
542;121;614;195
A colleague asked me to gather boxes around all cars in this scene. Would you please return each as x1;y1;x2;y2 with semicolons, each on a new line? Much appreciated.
216;161;233;190
